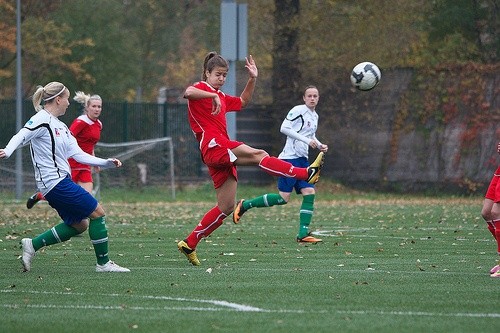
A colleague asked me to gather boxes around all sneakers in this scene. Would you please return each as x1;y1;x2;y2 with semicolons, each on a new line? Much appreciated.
307;152;325;184
27;193;40;209
296;232;322;242
232;199;247;224
490;265;500;273
177;240;200;266
95;260;130;272
20;238;35;271
490;270;500;278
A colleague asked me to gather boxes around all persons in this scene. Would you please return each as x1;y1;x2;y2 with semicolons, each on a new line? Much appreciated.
26;90;102;237
177;51;324;266
481;142;500;277
233;85;328;243
138;158;149;184
0;82;130;272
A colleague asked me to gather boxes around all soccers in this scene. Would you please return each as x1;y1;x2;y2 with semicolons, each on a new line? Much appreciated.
351;61;382;92
